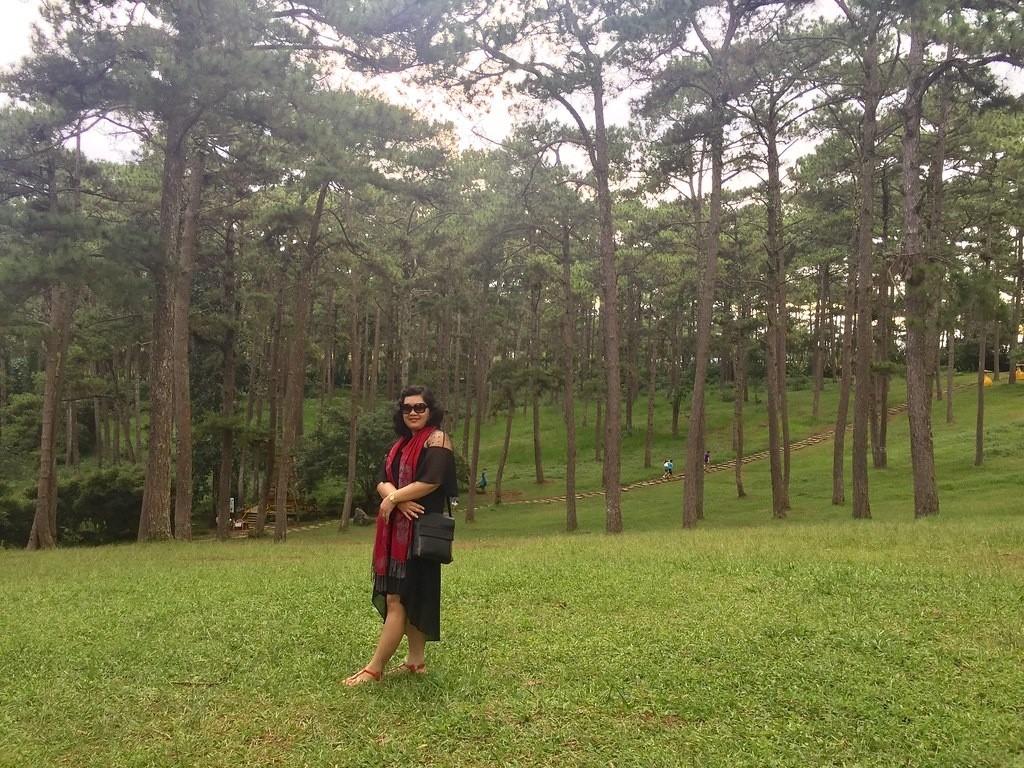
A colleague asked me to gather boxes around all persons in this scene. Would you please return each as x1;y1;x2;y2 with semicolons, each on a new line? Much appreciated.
339;383;459;688
663;460;674;480
704;451;710;467
451;496;460;511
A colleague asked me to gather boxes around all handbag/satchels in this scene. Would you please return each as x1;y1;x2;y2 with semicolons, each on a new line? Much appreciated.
413;512;455;564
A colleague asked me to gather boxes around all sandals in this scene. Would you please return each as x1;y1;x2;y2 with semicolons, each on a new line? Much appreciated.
341;669;381;687
383;663;428;676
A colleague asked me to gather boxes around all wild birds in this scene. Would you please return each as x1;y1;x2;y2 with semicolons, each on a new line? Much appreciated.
454;456;487;490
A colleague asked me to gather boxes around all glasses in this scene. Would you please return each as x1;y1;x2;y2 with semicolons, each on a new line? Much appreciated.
402;403;430;414
